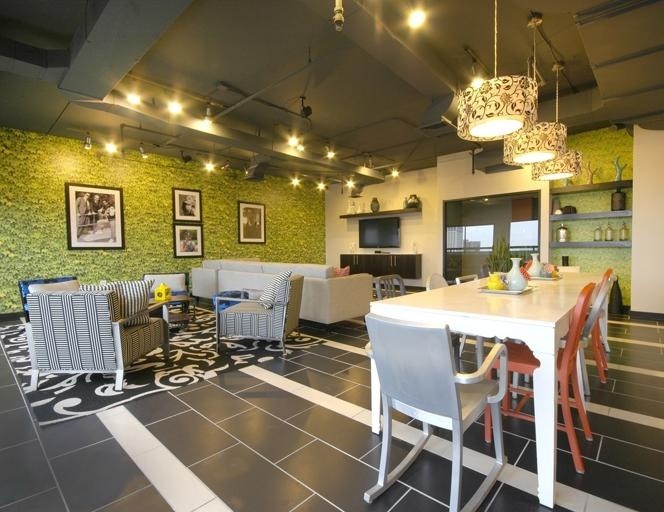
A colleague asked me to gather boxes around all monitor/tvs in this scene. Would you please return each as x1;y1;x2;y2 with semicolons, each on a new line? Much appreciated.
358;216;400;248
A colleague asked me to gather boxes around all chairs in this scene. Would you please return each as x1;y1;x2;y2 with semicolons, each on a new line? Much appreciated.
24;290;170;391
364;312;508;511
485;282;596;474
142;271;190;313
373;273;459;374
454;273;495;360
18;277;81;322
216;274;303;355
425;272;484;371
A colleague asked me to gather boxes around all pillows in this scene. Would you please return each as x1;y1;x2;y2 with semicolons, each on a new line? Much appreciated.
257;271;292;309
80;280;154;325
334;265;350;276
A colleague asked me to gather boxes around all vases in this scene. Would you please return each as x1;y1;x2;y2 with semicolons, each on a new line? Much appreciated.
408;194;419;208
402;196;408;208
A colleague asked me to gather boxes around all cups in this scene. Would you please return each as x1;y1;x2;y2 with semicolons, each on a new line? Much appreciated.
562;256;569;265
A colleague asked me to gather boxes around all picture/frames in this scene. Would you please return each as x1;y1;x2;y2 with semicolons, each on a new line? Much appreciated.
65;183;125;250
238;200;266;244
173;222;203;258
172;187;202;221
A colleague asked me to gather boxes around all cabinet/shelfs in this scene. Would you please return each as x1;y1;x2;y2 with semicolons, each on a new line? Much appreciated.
549;180;633;248
340;254;421;278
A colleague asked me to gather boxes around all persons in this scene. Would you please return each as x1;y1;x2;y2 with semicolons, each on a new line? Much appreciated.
243;208;261;238
185;201;196;216
76;193;115;240
180;232;197;252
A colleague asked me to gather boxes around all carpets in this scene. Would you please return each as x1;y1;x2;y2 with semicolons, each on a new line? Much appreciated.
0;307;324;427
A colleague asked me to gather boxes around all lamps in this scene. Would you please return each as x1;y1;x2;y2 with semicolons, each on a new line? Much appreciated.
456;0;537;141
502;14;567;167
530;62;582;182
333;0;345;31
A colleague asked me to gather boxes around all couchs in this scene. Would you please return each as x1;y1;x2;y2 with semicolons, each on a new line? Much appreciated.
190;260;373;337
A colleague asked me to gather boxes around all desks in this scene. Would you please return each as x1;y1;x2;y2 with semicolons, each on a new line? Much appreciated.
365;269;612;508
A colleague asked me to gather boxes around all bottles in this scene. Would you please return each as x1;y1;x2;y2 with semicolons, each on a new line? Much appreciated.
593;222;628;241
488;253;545;291
557;223;569;242
